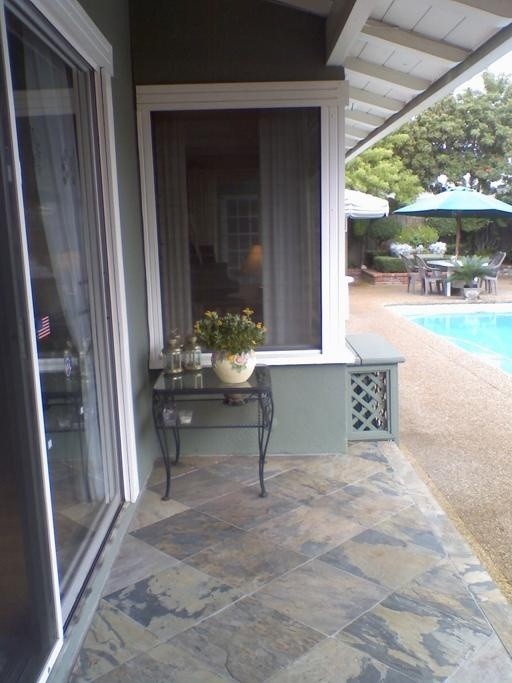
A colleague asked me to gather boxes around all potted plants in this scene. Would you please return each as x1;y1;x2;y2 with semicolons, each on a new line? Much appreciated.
444;253;492;301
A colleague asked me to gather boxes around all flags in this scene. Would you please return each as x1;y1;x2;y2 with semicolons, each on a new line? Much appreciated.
35;314;52;342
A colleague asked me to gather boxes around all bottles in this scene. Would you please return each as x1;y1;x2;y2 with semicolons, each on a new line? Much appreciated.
183;336;202;370
161;339;182;374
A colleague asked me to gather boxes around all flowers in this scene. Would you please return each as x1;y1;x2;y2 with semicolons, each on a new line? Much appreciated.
193;307;268;358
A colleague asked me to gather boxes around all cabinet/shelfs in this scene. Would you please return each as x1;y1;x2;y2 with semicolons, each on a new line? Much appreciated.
151;366;274;501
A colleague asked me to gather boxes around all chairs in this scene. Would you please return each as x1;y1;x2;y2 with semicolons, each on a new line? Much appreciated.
398;249;507;296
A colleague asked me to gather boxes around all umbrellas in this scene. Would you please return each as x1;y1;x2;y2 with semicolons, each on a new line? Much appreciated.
343;187;390;276
392;185;511;260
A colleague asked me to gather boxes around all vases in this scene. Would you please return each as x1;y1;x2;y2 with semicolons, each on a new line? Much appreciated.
210;345;257;384
